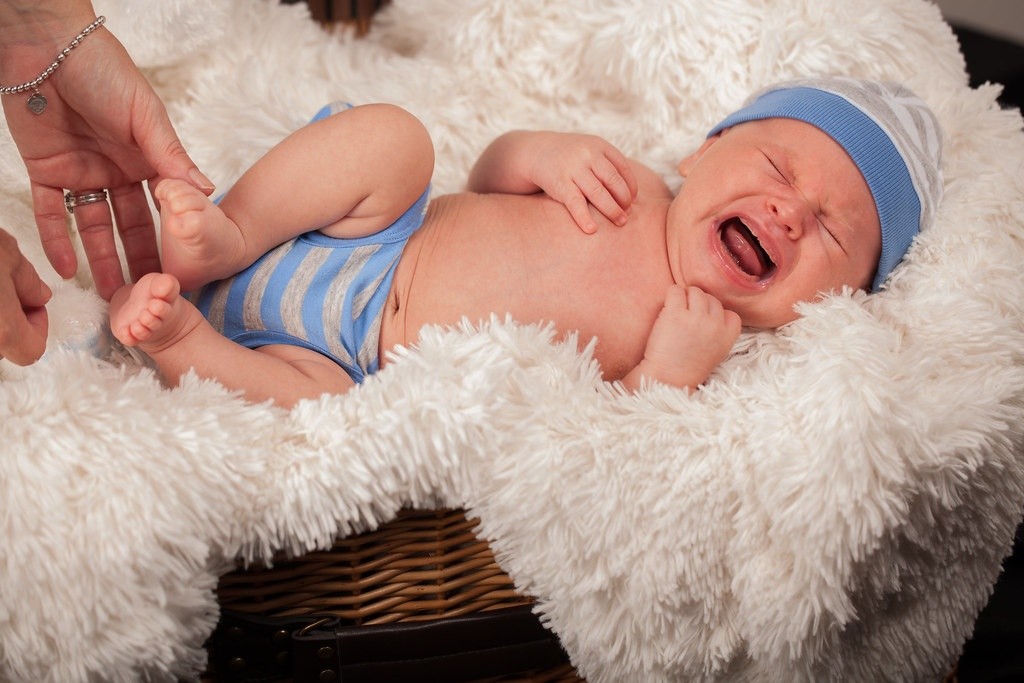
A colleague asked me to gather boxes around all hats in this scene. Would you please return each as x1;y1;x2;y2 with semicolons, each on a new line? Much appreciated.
705;76;944;299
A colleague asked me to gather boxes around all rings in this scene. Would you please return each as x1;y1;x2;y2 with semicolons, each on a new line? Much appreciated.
65;193;106;212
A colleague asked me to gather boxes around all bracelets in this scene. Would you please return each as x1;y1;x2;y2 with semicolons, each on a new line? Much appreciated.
0;15;105;114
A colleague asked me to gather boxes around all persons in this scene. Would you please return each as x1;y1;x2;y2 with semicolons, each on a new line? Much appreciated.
110;77;942;409
0;1;215;366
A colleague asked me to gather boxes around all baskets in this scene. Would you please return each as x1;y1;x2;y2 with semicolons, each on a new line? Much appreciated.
201;507;587;679
